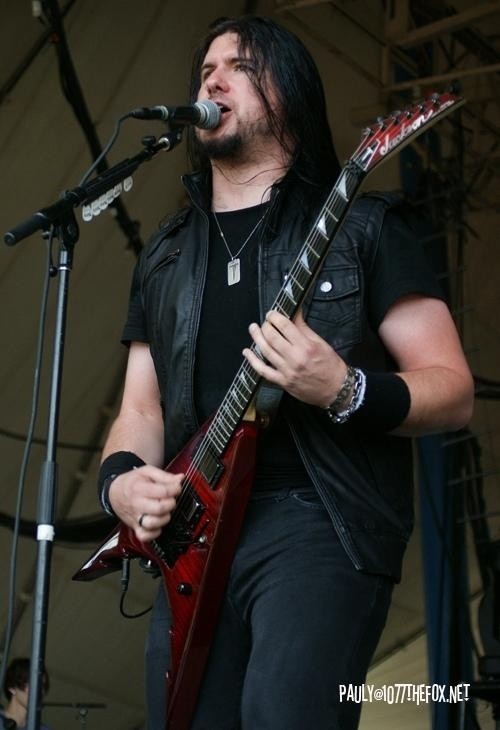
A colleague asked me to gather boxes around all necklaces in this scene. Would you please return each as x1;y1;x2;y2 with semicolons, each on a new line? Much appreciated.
211;206;271;286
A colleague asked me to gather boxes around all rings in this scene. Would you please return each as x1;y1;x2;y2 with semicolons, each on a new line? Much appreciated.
139;513;148;527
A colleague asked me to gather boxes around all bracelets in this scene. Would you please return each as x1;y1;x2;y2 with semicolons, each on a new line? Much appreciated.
97;451;147;519
320;366;411;434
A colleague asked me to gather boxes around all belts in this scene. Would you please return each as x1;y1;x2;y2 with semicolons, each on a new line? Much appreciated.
256;466;314;491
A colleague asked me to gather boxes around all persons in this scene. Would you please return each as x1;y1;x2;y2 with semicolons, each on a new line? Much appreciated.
97;16;475;730
0;657;49;730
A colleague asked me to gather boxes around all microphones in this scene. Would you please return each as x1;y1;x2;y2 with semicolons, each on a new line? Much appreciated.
129;99;221;130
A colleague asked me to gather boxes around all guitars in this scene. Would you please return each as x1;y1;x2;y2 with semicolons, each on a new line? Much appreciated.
70;86;467;729
